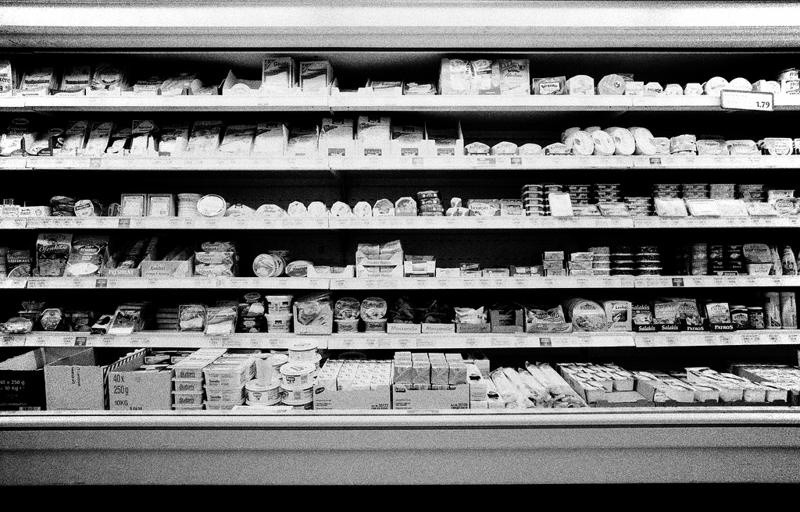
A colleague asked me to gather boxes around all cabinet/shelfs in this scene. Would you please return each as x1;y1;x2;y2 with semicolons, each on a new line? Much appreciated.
1;1;799;485
